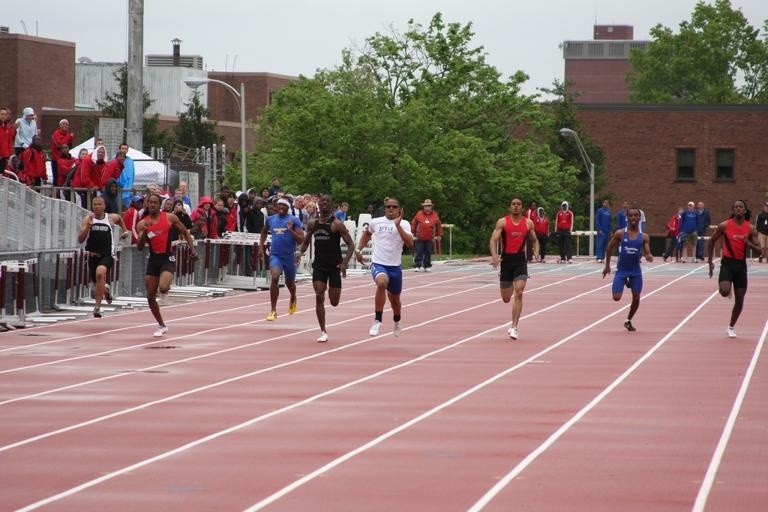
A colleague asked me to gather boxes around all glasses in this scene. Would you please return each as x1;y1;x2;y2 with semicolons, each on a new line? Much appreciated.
386;205;398;209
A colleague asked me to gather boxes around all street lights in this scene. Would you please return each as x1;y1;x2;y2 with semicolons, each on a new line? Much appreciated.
182;75;246;204
557;127;596;258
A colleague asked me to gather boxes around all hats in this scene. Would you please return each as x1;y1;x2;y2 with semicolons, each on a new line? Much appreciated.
422;199;433;205
198;197;215;207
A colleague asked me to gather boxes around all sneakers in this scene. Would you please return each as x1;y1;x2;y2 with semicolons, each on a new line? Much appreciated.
414;267;432;272
509;326;519;340
394;320;401;337
624;321;636;332
267;310;277;321
288;301;297;314
727;326;736;337
316;330;329;342
370;320;382;335
94;284;169;337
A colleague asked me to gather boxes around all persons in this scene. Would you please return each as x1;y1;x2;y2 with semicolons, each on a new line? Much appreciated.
78;197;128;317
525;202;575;264
409;199;443;272
117;181;374;342
356;197;413;337
1;107;136;216
594;199;768;338
489;198;540;339
